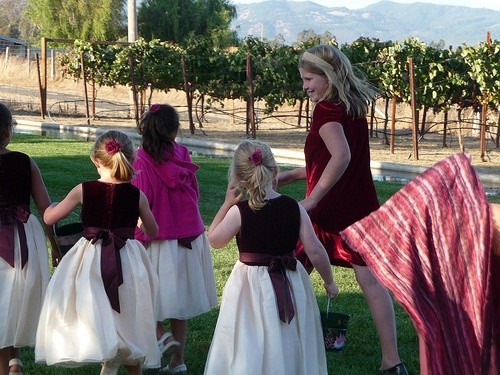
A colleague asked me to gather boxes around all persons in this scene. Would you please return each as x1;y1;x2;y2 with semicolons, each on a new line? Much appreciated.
124;102;219;375
32;128;164;375
0;101;63;375
201;139;339;375
271;42;411;375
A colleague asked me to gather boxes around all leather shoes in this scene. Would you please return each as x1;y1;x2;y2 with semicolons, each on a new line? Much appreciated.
378;363;409;375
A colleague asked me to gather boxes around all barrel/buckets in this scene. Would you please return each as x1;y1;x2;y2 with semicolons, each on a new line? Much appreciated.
320;293;349;351
53;212;84;258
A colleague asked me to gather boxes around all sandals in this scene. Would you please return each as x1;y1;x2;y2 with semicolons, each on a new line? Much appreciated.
157;361;187;373
8;358;24;375
156;331;181;356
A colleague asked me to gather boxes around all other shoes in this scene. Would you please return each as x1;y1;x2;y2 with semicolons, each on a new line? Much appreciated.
99;349;122;374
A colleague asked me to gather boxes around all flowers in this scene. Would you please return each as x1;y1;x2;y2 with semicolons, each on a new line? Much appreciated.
149;104;160;112
105;140;122;155
248;148;264;165
